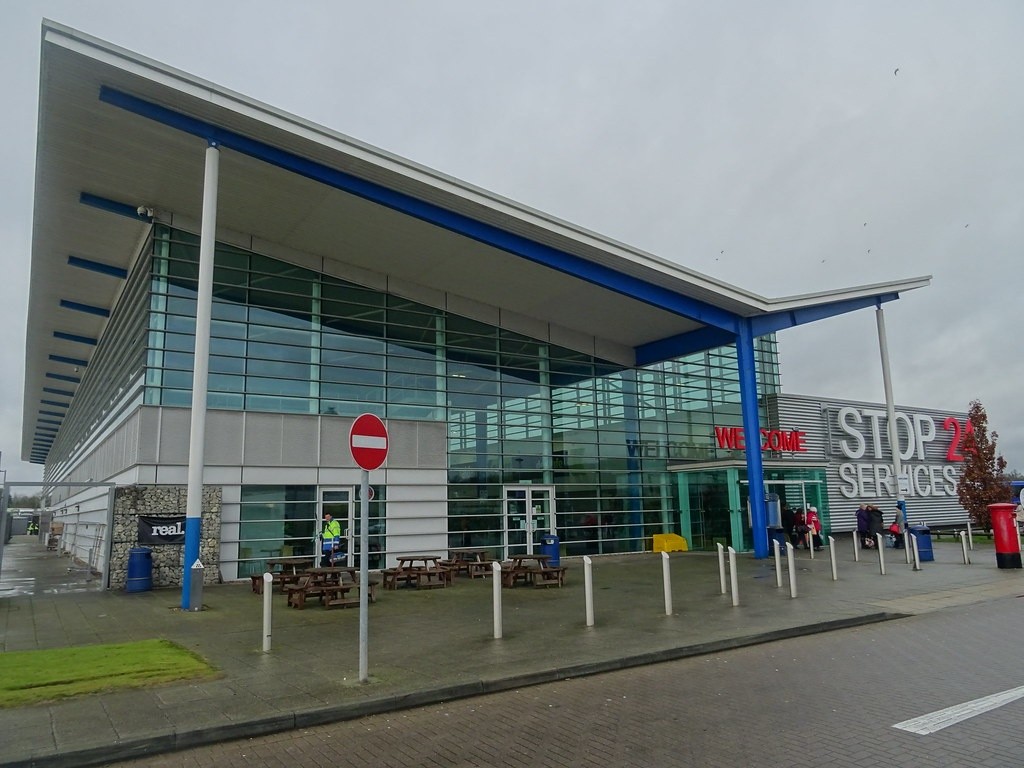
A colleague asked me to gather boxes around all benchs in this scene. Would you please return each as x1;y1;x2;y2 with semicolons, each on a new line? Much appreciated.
250;557;567;609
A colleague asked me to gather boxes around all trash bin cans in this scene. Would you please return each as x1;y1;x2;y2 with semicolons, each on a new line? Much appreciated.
908;525;934;562
540;535;561;568
125;547;153;593
768;528;786;557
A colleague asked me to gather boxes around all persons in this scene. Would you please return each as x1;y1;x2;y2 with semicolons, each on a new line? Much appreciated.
895;506;904;540
320;512;340;579
1015;502;1024;525
781;501;810;550
807;507;824;551
856;504;884;549
27;520;38;535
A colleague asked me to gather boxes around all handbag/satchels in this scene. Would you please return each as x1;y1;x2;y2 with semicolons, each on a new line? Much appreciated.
795;526;806;533
329;552;347;562
812;526;818;535
864;537;875;548
889;522;900;535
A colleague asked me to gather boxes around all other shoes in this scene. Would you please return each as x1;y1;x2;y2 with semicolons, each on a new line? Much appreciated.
794;545;800;549
814;548;823;551
804;547;809;549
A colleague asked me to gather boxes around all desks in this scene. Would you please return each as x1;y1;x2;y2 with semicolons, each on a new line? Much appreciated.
507;554;556;588
264;556;314;595
451;549;490;580
290;567;360;608
388;555;444;592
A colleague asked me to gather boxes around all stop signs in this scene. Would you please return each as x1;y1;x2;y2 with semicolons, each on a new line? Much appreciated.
349;413;389;473
